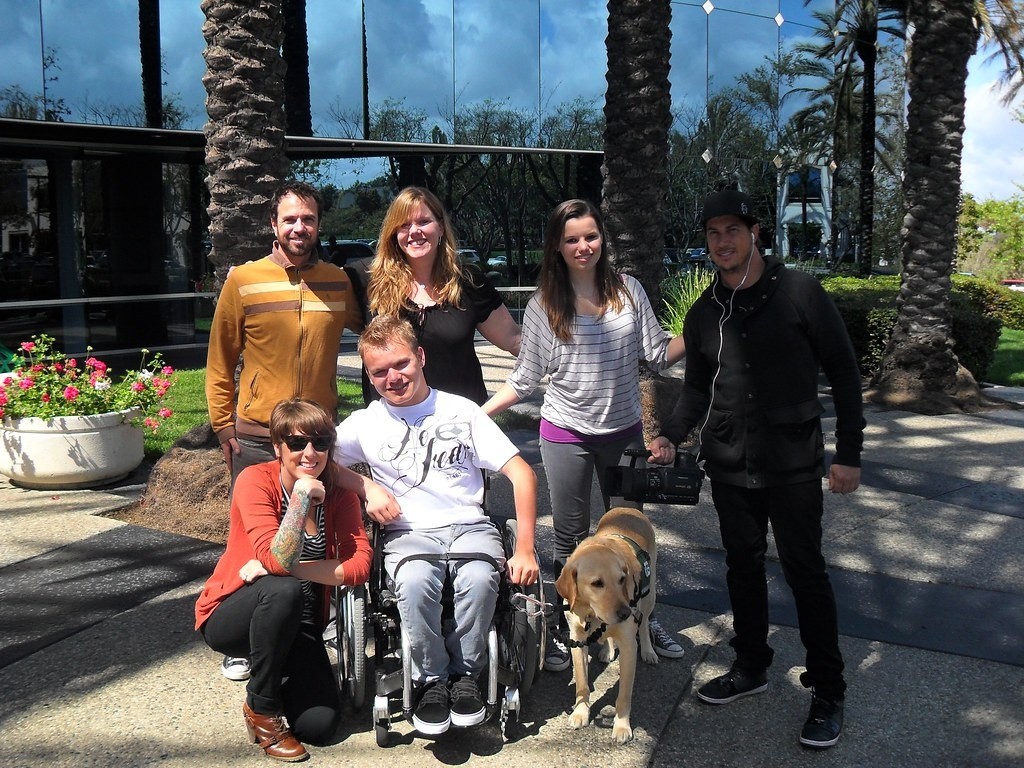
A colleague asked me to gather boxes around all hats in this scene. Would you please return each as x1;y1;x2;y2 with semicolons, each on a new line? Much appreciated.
699;190;767;228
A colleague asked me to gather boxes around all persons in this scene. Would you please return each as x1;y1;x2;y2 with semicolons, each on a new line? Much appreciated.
357;187;524;413
321;309;541;736
203;180;366;498
192;396;372;764
647;189;866;748
478;199;688;672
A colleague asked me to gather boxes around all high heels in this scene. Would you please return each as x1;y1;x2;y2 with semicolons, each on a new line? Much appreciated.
243;701;308;761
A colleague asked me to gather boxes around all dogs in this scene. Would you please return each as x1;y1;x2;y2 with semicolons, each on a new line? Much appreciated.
555;507;661;743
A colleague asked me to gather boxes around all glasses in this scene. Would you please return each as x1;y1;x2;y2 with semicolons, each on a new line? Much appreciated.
281;431;333;452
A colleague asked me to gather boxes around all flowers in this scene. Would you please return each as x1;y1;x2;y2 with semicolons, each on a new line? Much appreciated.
0;334;178;434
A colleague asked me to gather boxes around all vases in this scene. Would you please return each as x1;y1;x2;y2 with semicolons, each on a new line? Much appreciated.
0;406;144;489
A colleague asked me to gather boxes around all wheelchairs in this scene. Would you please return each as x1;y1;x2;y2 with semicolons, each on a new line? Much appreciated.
326;463;548;748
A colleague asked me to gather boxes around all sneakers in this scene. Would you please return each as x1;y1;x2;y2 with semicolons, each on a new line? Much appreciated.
696;660;768;702
543;631;571;671
221;654;252;680
450;675;486;726
636;620;685;657
413;675;452;735
800;690;844;746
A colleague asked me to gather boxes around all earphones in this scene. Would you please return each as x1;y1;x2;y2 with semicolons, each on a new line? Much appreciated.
752;232;755;243
